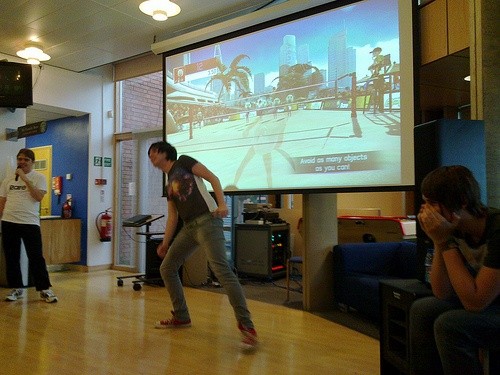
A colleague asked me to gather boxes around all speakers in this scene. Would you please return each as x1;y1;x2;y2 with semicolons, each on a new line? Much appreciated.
0;61;33;106
234;224;290;281
182;245;215;287
146;238;182;285
414;119;488;243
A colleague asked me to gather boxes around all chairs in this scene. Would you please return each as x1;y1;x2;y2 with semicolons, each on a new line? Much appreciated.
328;241;414;318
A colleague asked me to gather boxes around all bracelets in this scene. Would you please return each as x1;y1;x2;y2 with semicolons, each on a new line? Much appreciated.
439;238;458;251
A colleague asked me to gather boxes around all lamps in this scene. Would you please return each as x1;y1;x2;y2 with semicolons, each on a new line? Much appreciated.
16;45;52;65
139;0;181;22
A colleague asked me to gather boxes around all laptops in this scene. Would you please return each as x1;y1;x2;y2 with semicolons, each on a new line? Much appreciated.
122;214;151;225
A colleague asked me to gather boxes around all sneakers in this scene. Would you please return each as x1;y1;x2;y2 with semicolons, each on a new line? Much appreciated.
39;289;58;303
5;288;24;300
236;323;258;352
155;310;192;328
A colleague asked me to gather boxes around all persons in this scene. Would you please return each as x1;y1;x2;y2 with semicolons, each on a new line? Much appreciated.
148;141;258;349
274;98;280;118
410;165;500;375
286;94;293;116
245;102;251;122
259;100;264;117
166;103;228;127
0;148;57;303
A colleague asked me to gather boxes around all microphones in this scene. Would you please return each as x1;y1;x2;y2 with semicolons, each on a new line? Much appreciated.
15;164;22;181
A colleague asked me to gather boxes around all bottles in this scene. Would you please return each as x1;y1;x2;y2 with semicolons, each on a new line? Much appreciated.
425;250;436;283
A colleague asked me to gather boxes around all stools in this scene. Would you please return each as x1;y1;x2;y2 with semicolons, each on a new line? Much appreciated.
286;256;304;304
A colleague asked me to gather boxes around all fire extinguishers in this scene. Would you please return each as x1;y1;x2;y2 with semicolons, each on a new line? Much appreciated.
62;198;73;219
96;207;112;241
